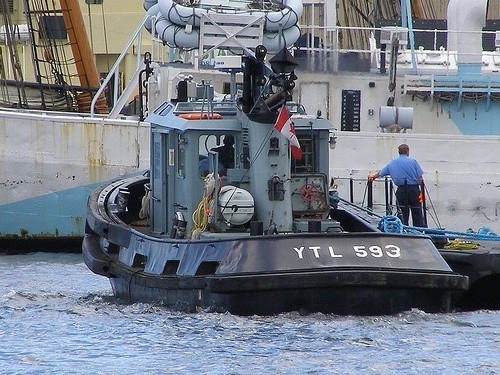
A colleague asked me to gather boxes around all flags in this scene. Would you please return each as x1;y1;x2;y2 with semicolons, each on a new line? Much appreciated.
275;105;302;160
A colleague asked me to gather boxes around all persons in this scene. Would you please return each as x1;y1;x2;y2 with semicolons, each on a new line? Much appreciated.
211;136;235;169
368;144;424;226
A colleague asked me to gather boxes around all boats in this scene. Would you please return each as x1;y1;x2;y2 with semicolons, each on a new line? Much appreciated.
0;0;499;247
82;0;499;315
